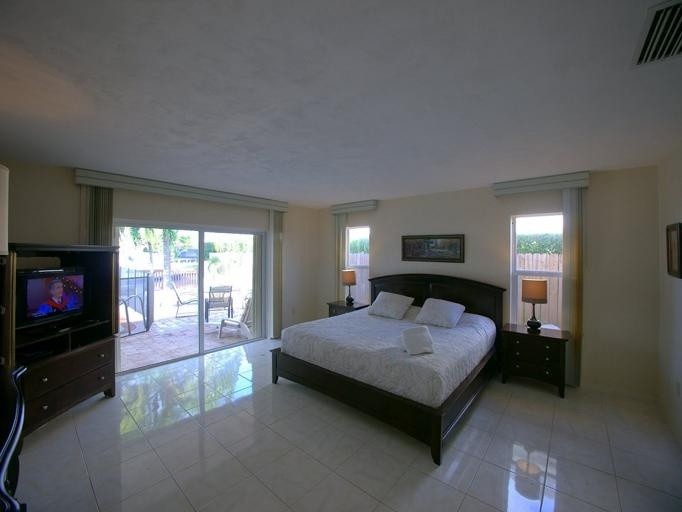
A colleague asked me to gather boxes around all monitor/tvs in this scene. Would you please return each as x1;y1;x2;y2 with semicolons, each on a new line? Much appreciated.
16;266;88;331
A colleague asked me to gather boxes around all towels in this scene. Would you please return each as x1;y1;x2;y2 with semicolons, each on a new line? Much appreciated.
398;325;437;356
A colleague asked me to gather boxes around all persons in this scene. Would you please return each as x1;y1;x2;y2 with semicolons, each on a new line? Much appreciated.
35;280;75;315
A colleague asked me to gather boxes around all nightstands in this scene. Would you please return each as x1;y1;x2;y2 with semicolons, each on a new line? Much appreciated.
325;299;370;318
496;323;573;400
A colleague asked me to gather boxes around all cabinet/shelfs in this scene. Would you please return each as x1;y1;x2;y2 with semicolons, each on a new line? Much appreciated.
7;242;121;439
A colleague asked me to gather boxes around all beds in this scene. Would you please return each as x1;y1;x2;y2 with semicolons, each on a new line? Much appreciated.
268;272;507;465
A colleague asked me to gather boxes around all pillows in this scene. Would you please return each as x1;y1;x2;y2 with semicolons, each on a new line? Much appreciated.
366;291;415;321
415;298;465;329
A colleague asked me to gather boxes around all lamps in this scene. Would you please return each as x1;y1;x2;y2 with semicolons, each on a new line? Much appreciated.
520;279;549;333
341;269;358;305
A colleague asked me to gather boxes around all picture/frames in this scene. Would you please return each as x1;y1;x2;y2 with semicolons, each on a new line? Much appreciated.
665;221;681;280
401;234;465;264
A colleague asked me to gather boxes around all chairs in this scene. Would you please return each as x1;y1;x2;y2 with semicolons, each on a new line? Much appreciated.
169;281;198;318
2;358;32;511
205;285;235;324
216;290;254;341
